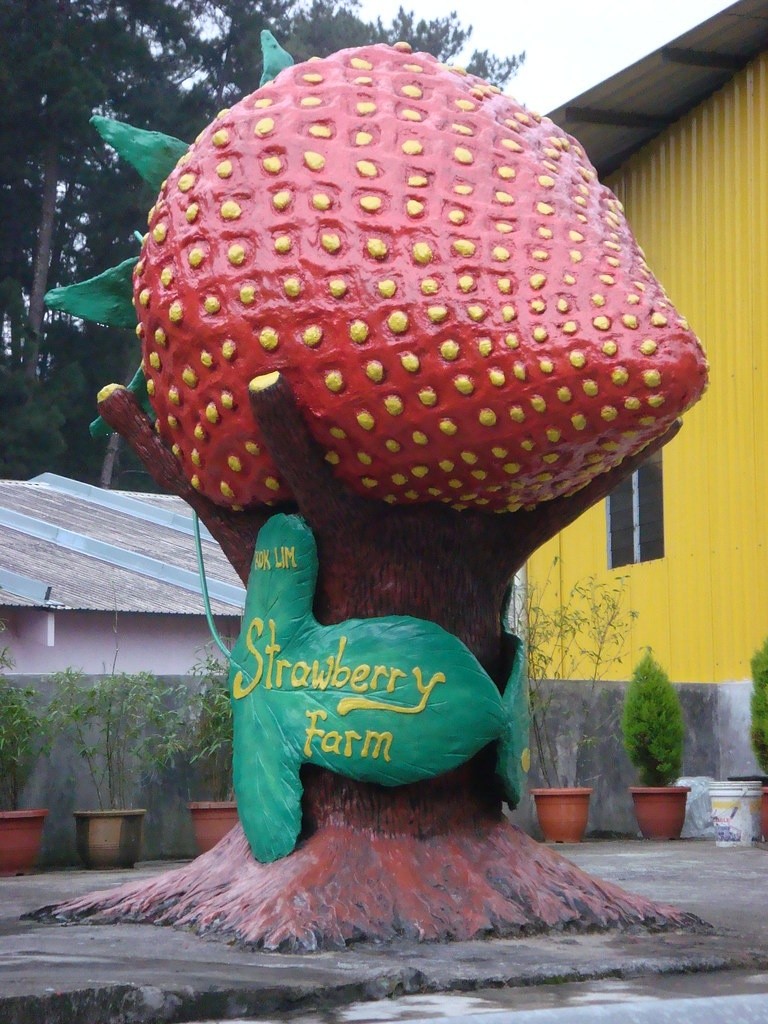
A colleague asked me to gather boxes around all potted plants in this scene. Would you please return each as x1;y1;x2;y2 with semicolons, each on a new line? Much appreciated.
748;642;768;842
623;648;688;842
44;663;190;871
505;556;649;844
186;636;250;851
0;672;47;877
